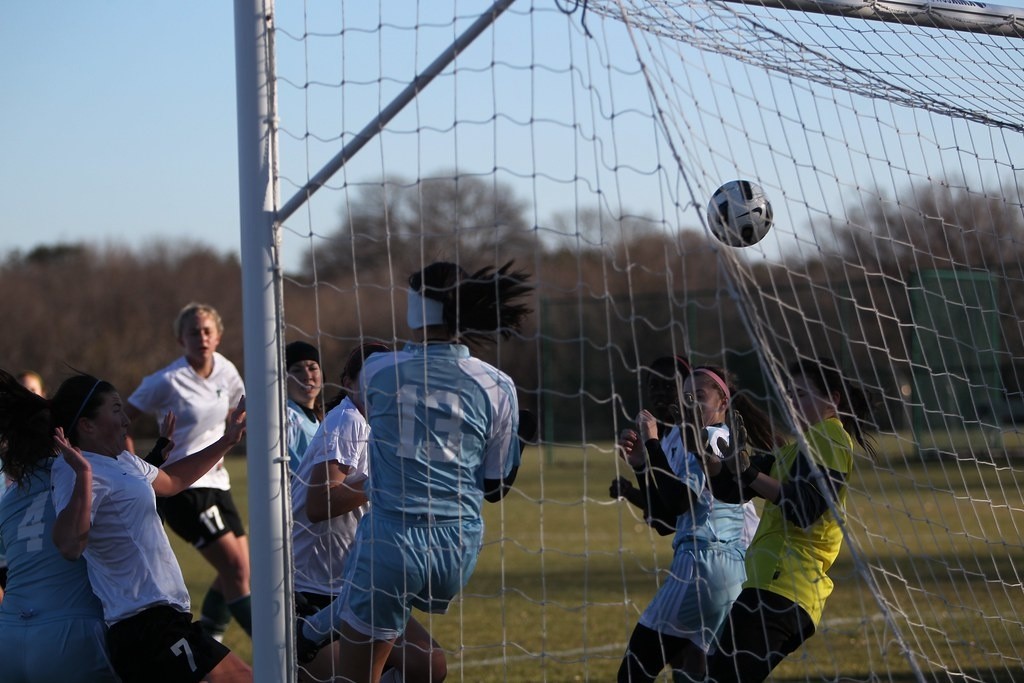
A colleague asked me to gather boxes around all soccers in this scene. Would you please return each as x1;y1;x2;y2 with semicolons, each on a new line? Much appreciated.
707;179;774;248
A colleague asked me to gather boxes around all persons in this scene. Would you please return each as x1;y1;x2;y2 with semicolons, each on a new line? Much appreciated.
297;261;538;683
0;402;177;683
285;339;327;474
0;368;254;683
291;342;447;683
609;355;692;536
616;365;788;683
124;302;252;643
669;358;880;683
15;370;44;397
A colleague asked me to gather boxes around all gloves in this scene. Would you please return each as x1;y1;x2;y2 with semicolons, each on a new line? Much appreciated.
716;410;760;487
669;392;712;461
